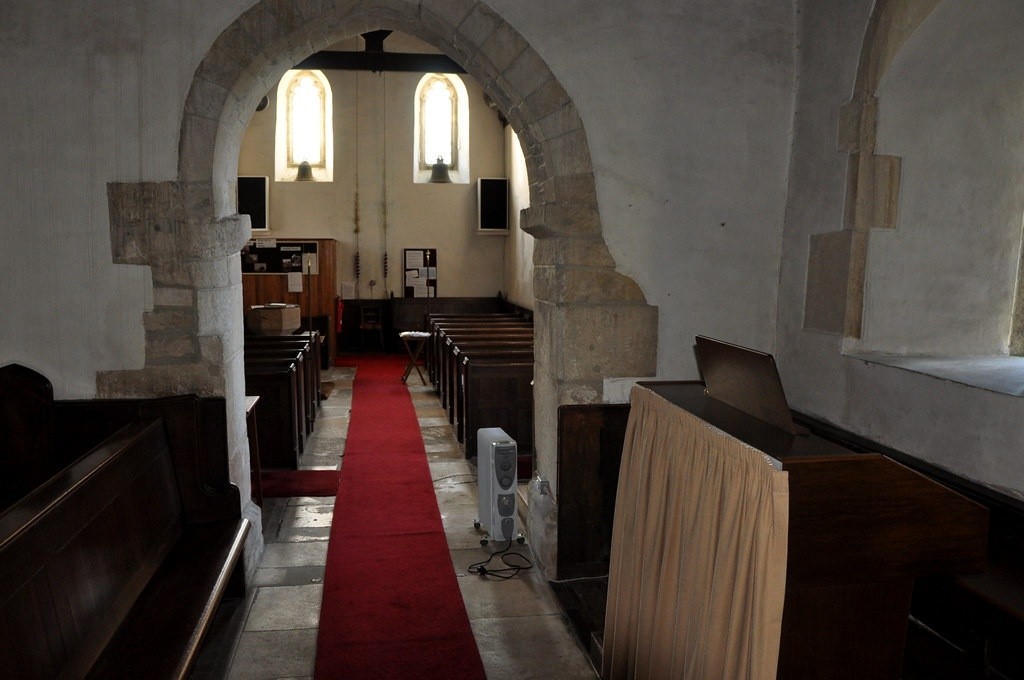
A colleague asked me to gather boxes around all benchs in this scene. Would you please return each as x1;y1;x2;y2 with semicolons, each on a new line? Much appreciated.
0;417;252;680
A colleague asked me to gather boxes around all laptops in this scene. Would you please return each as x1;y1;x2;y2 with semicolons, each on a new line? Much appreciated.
695;336;798;435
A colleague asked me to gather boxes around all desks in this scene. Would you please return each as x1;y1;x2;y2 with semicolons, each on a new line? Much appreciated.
400;331;432;387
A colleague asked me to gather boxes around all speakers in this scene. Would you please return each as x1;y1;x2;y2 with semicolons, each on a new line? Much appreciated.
476;177;510;231
237;176;269;231
